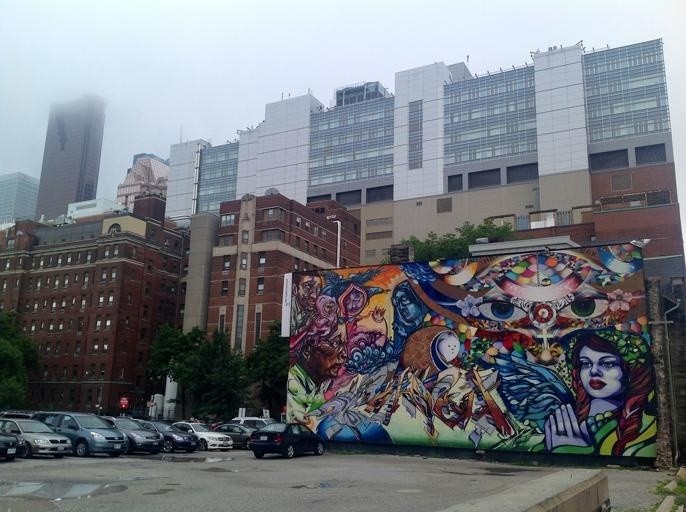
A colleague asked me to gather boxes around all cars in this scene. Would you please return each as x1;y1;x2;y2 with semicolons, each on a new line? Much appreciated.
249;424;324;458
0;411;278;459
